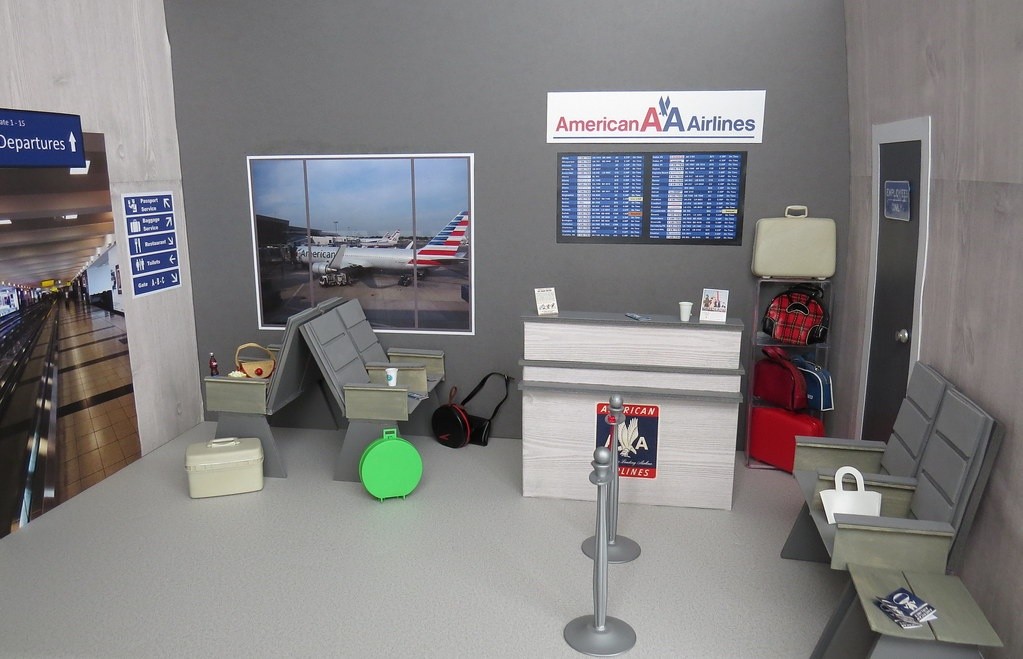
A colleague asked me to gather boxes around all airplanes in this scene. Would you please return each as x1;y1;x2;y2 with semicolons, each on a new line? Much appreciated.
288;209;470;287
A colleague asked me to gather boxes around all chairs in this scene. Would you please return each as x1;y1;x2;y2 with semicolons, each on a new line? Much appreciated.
201;294;449;483
780;359;1007;659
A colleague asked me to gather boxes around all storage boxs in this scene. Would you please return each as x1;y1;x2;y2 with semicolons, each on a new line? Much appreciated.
182;436;264;499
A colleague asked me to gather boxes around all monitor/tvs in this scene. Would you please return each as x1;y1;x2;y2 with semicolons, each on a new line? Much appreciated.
557;151;746;245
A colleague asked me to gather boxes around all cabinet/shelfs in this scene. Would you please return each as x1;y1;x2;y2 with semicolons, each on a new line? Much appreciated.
745;274;837;472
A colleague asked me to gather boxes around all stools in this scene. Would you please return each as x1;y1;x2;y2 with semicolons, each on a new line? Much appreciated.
820;561;1006;659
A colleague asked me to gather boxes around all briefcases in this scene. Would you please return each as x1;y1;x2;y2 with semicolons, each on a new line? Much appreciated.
751;408;824;473
750;204;836;280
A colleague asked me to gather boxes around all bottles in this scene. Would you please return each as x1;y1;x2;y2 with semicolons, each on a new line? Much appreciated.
209;353;219;377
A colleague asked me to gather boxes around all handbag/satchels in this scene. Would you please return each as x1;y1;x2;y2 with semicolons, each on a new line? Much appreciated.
432;386;470;448
461;374;508;448
753;349;834;411
820;465;882;526
235;344;276;380
762;283;830;346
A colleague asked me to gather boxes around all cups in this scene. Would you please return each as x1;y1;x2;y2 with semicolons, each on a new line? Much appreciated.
385;368;399;387
679;302;693;322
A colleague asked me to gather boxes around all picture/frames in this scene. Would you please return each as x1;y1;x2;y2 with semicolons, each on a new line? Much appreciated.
246;152;476;337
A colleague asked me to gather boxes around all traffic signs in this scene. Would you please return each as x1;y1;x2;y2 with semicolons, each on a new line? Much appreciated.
0;107;87;167
121;189;182;298
